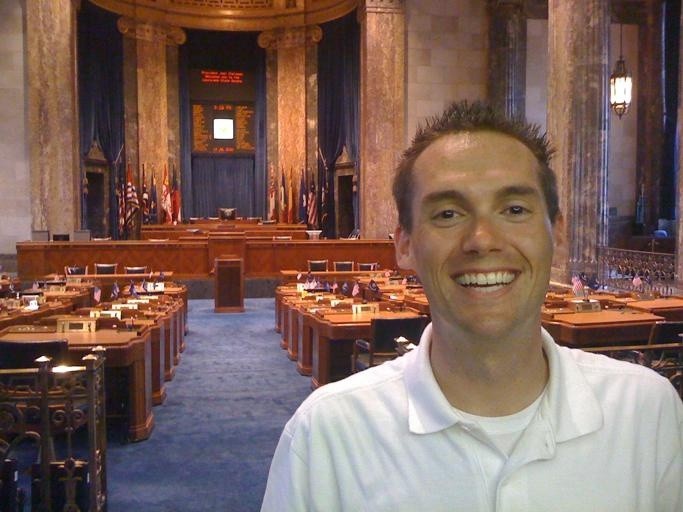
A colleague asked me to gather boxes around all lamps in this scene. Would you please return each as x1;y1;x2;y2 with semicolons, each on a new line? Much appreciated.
608;0;633;121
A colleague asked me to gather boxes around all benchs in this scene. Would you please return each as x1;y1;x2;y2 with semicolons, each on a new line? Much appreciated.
14;207;417;280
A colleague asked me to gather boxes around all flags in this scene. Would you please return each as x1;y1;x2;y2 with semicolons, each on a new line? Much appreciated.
297;271;379;300
572;271;651;296
93;271;165;303
269;160;329;237
116;161;181;240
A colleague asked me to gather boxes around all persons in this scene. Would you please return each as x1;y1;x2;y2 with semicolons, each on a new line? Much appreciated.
260;99;683;512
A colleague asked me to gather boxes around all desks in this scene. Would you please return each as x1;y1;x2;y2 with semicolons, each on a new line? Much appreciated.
272;257;683;393
0;263;188;510
